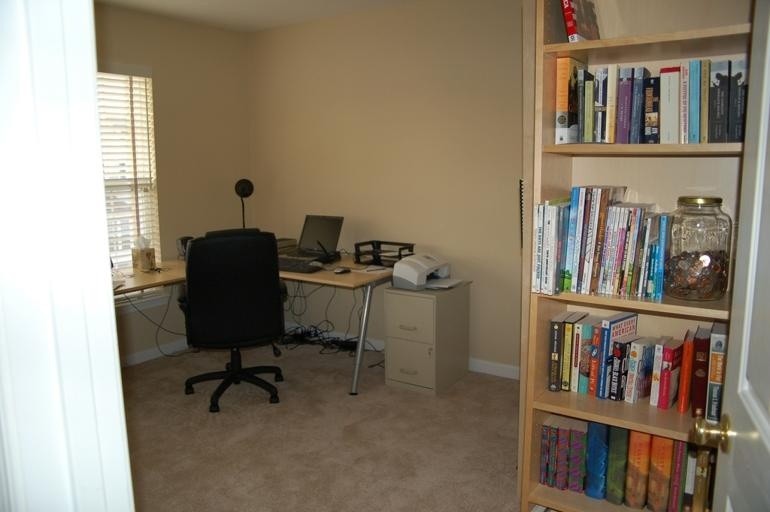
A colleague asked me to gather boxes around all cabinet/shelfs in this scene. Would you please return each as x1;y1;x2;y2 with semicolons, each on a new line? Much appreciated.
383;283;471;397
516;1;755;512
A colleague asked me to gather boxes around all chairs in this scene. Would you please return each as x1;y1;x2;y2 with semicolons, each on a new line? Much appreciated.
177;225;291;414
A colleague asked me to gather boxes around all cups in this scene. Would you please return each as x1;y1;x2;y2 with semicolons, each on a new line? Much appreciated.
177;236;194;258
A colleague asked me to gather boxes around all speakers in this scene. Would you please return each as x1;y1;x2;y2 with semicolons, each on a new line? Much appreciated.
176;236;194;261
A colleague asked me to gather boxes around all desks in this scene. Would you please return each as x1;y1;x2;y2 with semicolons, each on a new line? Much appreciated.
111;253;394;399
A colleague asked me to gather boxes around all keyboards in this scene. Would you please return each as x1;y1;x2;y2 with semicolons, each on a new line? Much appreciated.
277;257;321;273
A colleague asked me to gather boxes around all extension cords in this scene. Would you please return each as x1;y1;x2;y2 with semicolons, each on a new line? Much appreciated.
303;335;324;341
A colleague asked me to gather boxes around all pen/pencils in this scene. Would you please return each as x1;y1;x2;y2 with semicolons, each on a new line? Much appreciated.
367;268;386;272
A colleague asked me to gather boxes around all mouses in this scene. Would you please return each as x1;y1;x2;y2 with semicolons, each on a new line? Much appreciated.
335;267;350;274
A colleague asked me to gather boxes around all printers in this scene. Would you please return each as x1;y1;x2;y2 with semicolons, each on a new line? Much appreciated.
393;251;452;292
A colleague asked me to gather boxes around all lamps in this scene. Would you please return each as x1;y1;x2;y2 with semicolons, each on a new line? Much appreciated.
234;179;254;227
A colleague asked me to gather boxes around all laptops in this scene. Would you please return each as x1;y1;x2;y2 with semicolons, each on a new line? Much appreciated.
277;215;344;263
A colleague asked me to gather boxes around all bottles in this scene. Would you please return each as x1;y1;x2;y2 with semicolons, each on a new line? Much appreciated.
663;194;730;302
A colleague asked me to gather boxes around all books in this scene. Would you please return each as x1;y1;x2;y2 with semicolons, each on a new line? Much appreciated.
531;186;671;302
554;54;748;149
561;0;602;43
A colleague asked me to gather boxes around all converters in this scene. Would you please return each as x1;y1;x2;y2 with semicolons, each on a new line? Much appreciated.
342;341;357;348
294;335;303;340
